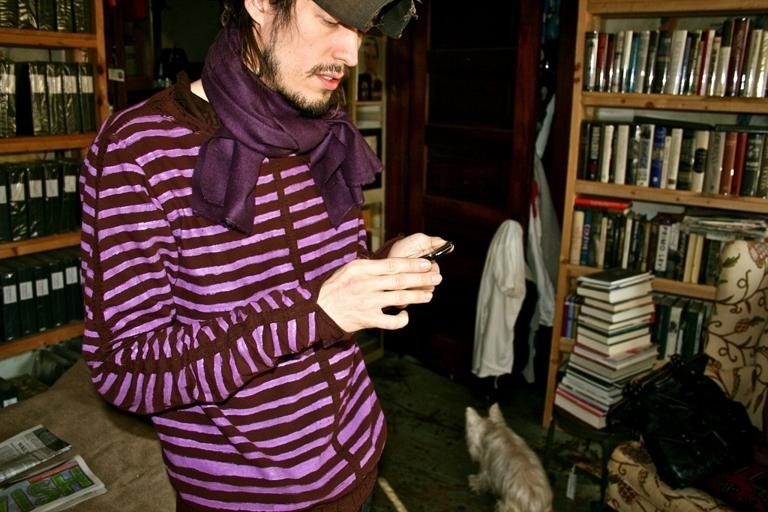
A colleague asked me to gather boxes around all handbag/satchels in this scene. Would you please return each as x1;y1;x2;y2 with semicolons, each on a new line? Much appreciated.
621;353;756;490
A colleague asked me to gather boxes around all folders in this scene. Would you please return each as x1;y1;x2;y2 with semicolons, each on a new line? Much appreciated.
0;58;97;345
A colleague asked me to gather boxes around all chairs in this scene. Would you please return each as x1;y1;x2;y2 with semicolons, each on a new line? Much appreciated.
600;240;768;511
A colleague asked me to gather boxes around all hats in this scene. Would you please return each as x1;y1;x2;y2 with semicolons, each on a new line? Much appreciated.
312;0;418;40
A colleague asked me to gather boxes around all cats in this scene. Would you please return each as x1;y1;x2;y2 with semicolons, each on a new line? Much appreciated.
465;403;554;512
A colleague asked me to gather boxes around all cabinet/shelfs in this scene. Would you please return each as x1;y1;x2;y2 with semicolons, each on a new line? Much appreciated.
0;1;109;406
540;0;768;430
344;32;388;365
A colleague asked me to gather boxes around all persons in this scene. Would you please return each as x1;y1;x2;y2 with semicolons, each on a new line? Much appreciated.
79;0;456;512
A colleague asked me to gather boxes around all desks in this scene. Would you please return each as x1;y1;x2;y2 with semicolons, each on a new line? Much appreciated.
542;403;621;512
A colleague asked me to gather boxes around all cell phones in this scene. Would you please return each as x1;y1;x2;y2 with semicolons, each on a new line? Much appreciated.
407;241;451;261
